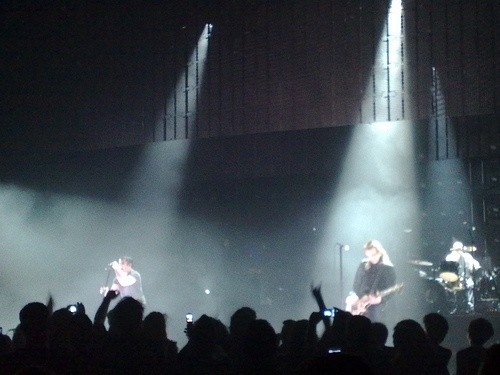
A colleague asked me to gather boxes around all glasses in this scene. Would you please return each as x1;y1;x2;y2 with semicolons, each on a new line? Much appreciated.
119;261;127;265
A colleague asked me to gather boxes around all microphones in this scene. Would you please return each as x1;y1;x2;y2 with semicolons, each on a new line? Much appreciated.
336;242;349;251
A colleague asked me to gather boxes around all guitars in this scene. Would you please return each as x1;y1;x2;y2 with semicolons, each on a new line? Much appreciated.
346;284;403;315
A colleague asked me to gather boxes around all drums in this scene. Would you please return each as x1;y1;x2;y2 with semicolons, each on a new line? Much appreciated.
439;261;460;282
418;278;458;314
477;277;499;302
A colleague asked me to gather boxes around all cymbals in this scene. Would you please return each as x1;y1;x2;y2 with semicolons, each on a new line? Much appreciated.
411;260;433;266
450;246;477;252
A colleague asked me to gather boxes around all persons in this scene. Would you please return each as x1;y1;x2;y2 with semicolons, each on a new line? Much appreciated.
349;239;396;325
0;280;500;375
100;259;146;306
446;242;480;313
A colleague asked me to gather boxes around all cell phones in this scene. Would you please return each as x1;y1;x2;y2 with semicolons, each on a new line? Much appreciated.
185;314;193;326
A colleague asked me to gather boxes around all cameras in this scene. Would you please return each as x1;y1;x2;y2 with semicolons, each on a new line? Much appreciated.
323;307;337;318
68;305;76;313
